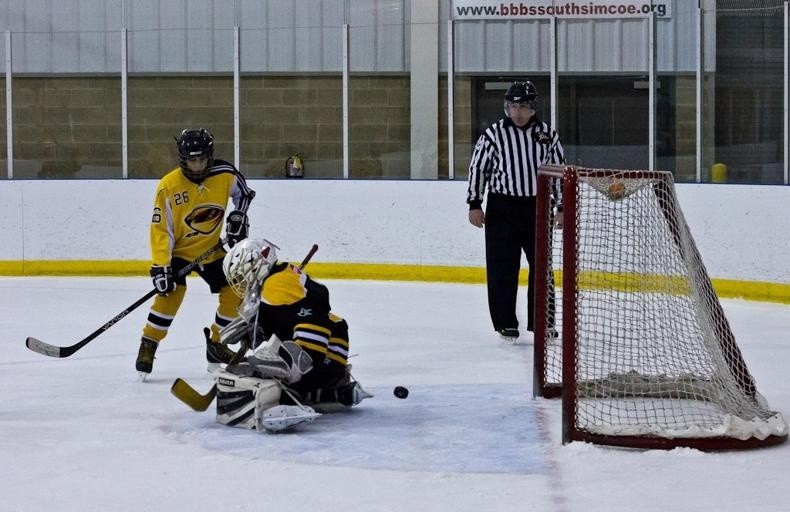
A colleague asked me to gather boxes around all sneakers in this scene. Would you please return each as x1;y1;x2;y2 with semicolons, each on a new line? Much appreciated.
261;405;316;432
346;381;362;406
535;326;559;338
499;327;521;337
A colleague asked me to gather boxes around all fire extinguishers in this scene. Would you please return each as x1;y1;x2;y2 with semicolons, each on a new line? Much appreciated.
286;153;304;179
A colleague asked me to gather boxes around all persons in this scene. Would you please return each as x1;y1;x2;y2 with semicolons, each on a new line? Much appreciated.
216;237;373;434
467;80;567;336
136;128;258;373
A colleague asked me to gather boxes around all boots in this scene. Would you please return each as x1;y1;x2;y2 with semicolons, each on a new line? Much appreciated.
135;337;158;373
204;326;247;364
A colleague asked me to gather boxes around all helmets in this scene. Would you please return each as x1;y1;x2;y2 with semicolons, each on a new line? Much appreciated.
221;238;278;297
175;127;215;180
504;80;539;118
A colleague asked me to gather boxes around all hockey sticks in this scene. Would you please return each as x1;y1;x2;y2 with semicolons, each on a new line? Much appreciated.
25;238;228;357
172;245;319;411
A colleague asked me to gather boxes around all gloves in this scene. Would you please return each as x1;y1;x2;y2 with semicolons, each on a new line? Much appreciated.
225;210;250;244
149;266;177;298
